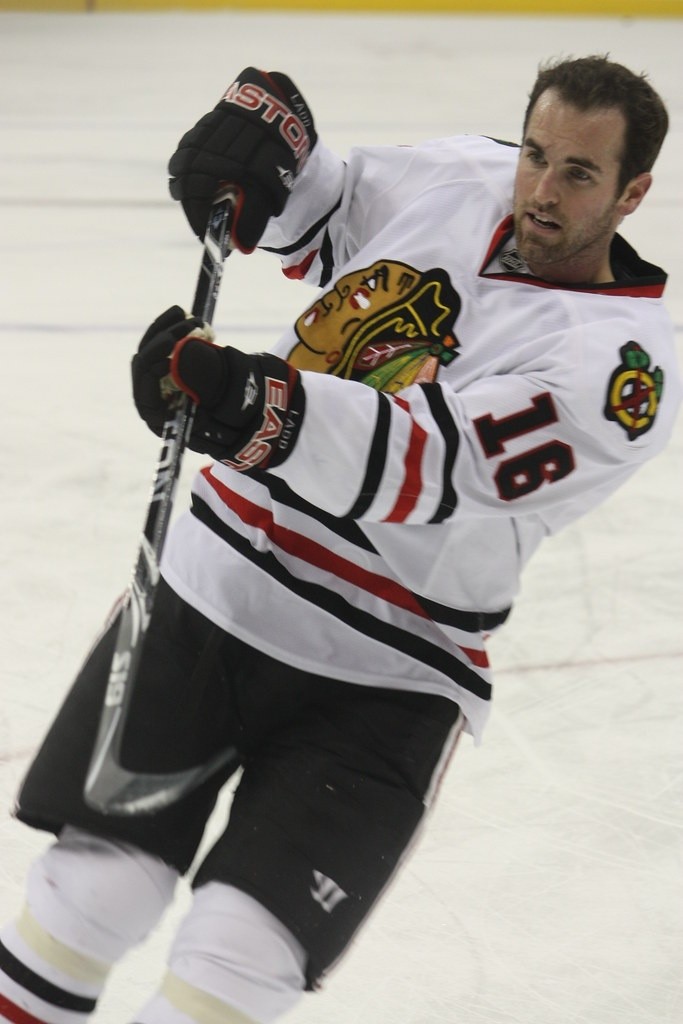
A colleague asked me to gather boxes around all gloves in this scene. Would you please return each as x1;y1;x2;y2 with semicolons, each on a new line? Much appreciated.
168;67;320;256
131;306;302;475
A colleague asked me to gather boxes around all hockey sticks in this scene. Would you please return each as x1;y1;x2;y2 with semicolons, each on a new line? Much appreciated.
80;182;242;817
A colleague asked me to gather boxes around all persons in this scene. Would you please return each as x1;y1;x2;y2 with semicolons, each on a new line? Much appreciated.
0;55;676;1024
